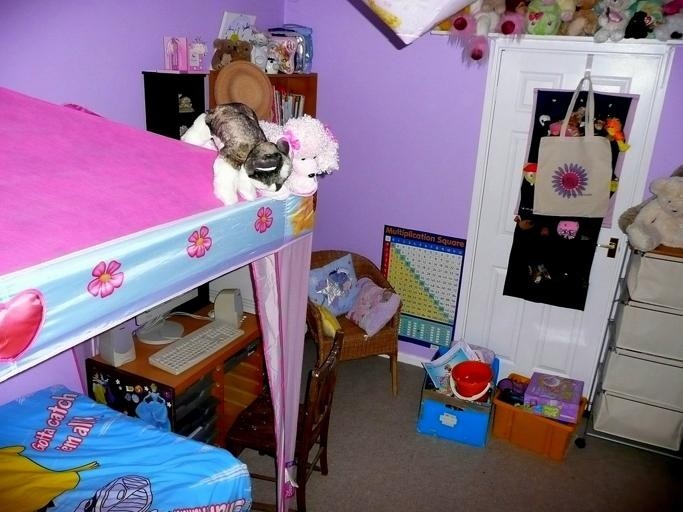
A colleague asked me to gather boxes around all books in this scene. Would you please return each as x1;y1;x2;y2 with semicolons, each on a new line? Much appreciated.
270;85;305;126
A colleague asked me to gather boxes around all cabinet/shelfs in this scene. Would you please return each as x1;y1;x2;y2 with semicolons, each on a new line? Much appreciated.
141;67;317;317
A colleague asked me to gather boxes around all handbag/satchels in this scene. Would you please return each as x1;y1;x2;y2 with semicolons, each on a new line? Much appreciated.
532;78;612;219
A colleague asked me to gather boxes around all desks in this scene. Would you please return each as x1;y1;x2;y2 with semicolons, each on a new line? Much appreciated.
86;301;269;451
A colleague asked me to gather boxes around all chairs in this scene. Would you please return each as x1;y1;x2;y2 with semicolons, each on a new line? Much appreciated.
307;250;403;397
225;328;344;512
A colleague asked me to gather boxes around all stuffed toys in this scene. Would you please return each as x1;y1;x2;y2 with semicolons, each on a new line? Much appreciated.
549;107;586;138
179;103;292;206
618;165;683;235
626;176;683;254
212;39;252;71
605;118;630;152
451;0;682;67
259;114;339;196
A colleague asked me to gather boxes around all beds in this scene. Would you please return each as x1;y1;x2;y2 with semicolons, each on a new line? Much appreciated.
0;86;316;510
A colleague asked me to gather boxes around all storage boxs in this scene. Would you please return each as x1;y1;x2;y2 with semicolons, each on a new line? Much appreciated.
589;241;683;455
493;372;588;462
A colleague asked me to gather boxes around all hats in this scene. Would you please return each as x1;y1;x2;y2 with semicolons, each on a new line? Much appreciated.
212;60;275;121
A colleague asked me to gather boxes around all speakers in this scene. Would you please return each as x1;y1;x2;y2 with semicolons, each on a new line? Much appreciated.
99;324;136;365
209;289;244;329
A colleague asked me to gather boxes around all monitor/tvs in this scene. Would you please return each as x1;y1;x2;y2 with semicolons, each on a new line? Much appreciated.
134;289;199;346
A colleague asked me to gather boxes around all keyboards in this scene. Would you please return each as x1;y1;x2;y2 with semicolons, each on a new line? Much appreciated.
149;319;246;376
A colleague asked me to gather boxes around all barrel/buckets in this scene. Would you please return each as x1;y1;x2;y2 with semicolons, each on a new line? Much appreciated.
448;359;494;403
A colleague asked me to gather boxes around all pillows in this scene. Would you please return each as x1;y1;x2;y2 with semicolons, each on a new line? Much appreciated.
345;277;401;338
308;254;358;317
